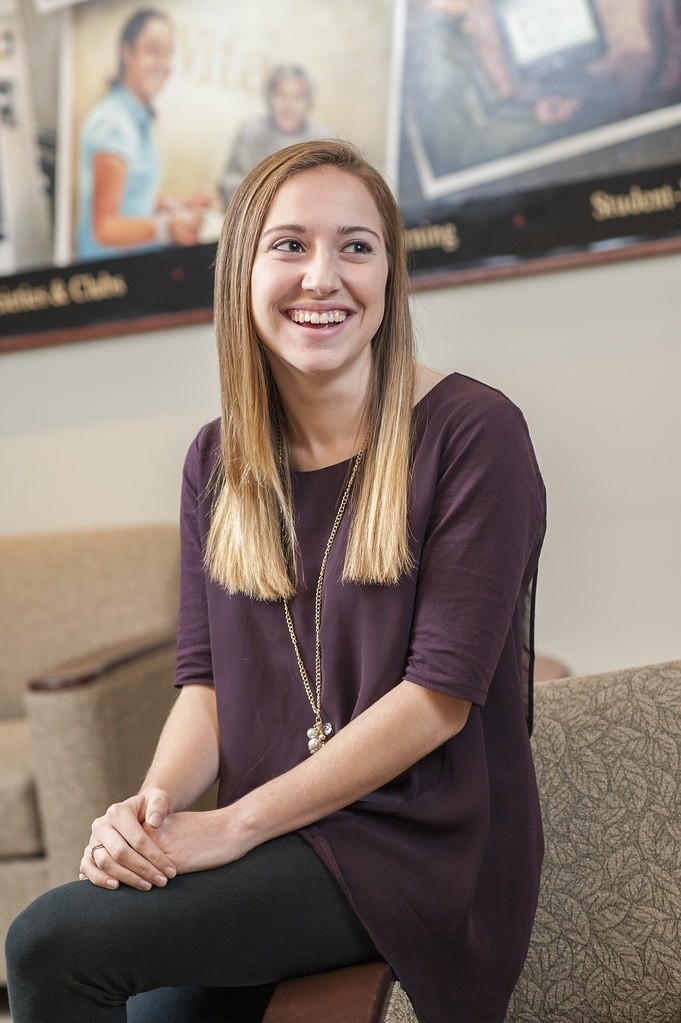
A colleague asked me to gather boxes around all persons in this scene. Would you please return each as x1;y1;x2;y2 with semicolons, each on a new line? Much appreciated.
5;140;547;1023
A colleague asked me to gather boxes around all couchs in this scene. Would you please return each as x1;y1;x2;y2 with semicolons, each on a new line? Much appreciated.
264;659;681;1019
0;524;219;985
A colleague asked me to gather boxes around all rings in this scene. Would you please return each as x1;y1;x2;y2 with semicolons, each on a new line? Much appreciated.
91;844;105;861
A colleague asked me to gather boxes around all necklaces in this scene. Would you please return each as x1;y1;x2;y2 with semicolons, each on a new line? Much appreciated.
276;413;377;758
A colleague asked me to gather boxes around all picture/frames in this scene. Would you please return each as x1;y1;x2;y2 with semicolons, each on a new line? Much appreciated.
405;0;681;201
53;0;407;268
